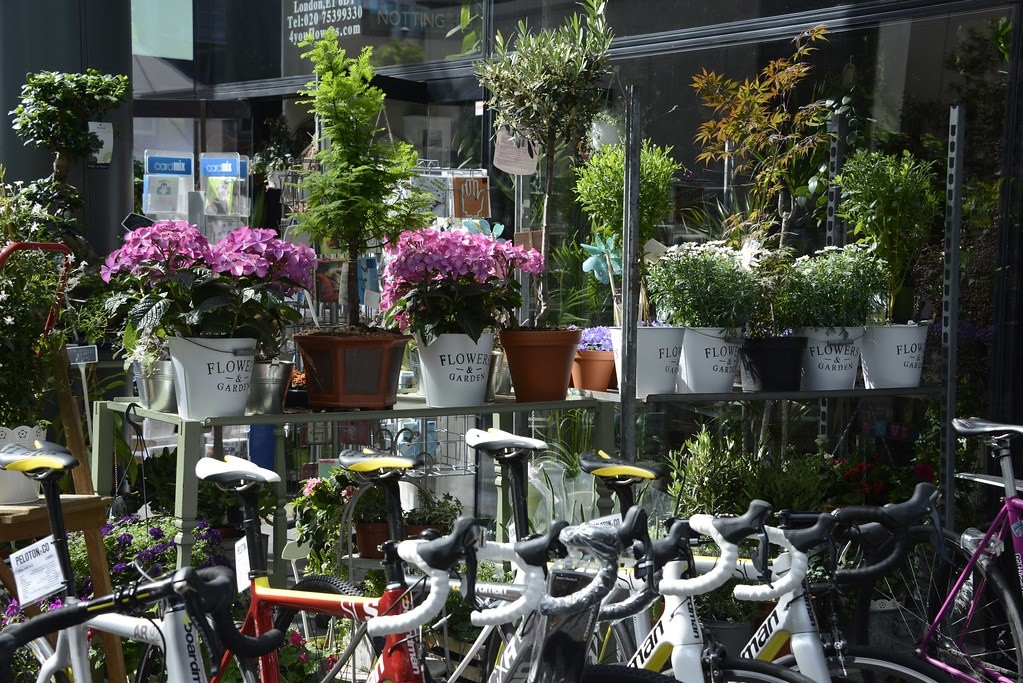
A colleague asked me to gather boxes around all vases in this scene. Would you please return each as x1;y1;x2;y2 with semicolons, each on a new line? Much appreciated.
164;336;256;421
412;331;494;407
572;352;614;392
358;519;398;560
824;504;865;548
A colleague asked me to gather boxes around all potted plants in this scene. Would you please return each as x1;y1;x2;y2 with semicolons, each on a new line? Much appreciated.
0;1;1023;683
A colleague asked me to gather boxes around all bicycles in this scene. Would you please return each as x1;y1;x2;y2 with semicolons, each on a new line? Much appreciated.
0;415;1022;683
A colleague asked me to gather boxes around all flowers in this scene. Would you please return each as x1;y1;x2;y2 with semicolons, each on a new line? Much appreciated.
293;470;399;546
100;220;319;342
380;228;545;344
0;513;337;682
576;325;614;352
828;449;937;506
636;319;683;328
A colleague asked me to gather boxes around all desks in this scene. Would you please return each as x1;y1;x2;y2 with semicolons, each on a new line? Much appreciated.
0;494;127;683
